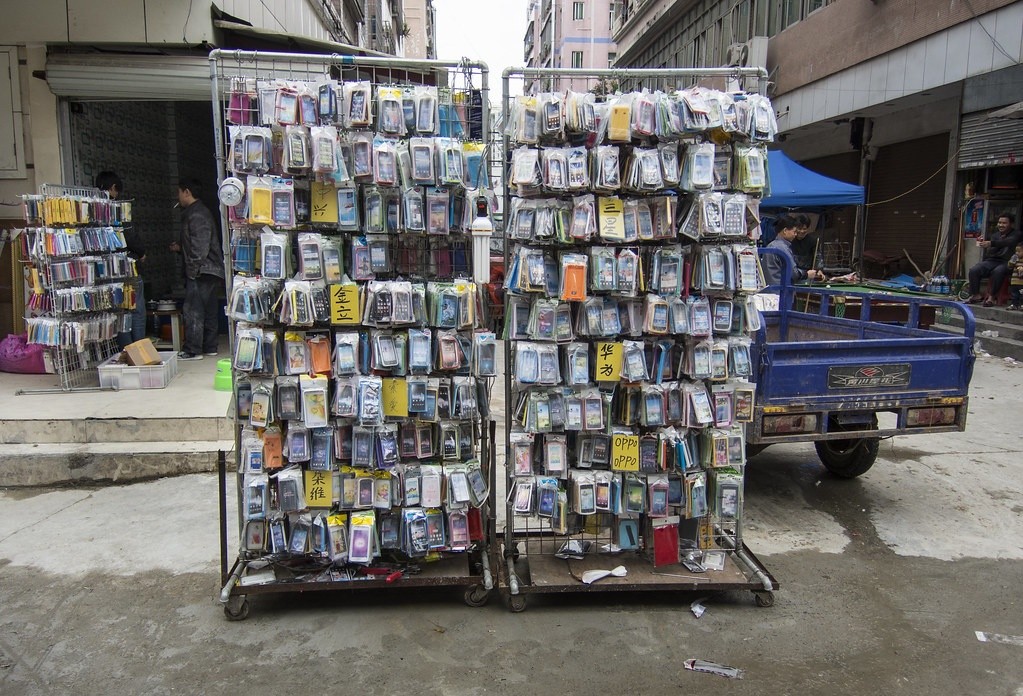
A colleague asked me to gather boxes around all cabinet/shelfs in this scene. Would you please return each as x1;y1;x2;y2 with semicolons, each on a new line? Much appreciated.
12;183;138;396
211;48;780;620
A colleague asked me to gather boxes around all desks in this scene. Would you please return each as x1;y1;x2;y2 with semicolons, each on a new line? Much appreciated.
795;284;957;332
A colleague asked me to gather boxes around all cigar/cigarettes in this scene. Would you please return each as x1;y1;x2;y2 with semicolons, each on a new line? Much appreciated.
174;202;179;208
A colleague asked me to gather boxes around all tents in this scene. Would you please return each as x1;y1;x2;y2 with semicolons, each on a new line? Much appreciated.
759;150;864;260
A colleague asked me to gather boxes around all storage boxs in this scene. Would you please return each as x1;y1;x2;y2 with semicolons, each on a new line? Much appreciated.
96;351;178;390
124;338;161;366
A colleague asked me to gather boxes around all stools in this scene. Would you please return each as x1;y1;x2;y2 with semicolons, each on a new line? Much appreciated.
147;310;183;355
984;275;1009;306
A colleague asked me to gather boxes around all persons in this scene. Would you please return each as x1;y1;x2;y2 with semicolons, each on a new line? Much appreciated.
789;214;825;284
962;213;1022;307
762;216;817;311
169;179;225;361
1006;242;1023;310
96;171;146;352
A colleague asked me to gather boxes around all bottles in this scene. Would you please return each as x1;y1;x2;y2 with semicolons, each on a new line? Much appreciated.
926;276;950;294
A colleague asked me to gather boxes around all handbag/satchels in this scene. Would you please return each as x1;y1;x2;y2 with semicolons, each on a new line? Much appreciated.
0;330;51;374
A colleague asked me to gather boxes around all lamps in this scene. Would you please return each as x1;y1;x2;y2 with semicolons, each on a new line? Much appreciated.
863;144;872;160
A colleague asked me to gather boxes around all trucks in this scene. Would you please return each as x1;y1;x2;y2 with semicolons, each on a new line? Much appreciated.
742;247;977;483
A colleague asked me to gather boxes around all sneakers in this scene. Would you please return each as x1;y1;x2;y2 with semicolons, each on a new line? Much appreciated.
178;351;204;360
204;352;219;356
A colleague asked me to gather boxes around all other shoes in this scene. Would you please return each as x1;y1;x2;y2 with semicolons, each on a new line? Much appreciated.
1019;305;1023;310
983;300;994;307
1006;304;1018;310
963;296;980;304
